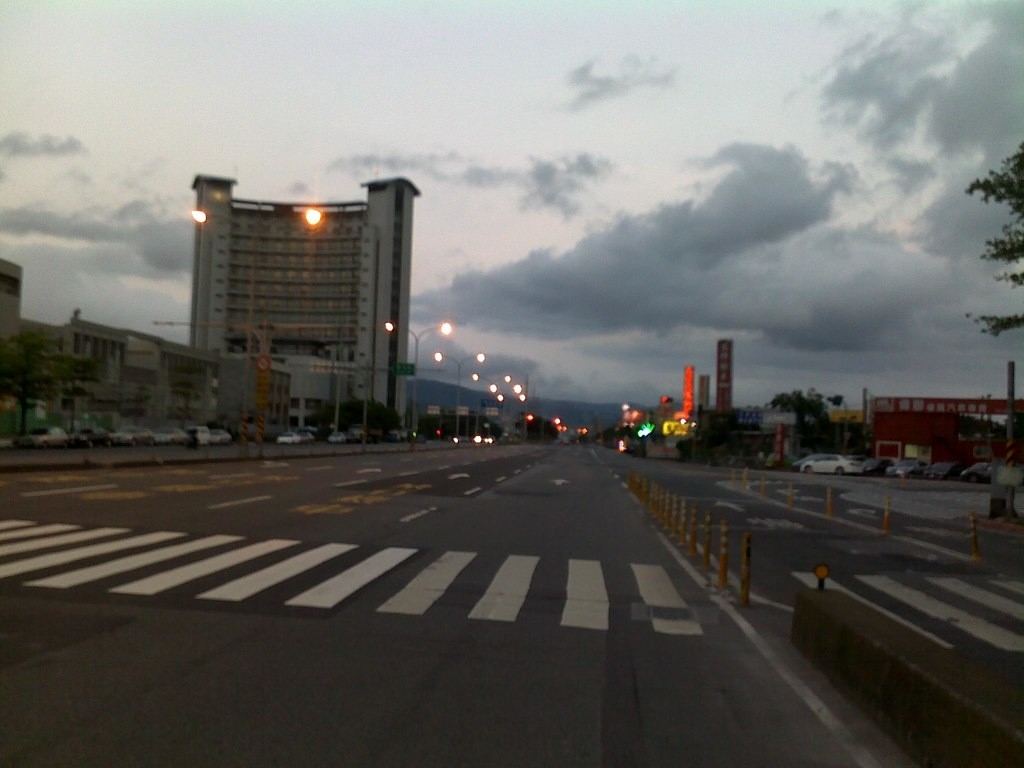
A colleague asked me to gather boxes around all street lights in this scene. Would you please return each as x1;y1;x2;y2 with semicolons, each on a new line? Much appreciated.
434;353;485;444
472;373;511;437
385;323;451;445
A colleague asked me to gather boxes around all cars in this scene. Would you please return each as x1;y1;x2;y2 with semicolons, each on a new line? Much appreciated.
70;429;111;448
278;431;316;445
112;426;158;447
799;453;865;476
960;463;993;483
792;455;816;471
208;430;232;444
12;426;69;449
185;426;210;447
152;427;188;445
922;462;968;481
848;454;867;461
885;459;928;479
863;457;893;474
329;428;366;443
